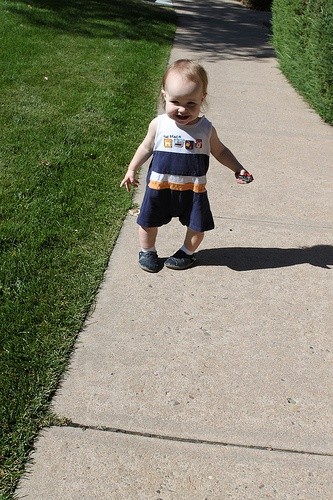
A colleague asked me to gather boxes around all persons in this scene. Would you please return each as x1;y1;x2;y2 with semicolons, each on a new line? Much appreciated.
119;57;254;275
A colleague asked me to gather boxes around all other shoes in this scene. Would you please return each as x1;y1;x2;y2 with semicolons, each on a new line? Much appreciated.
164;249;197;270
139;250;159;273
